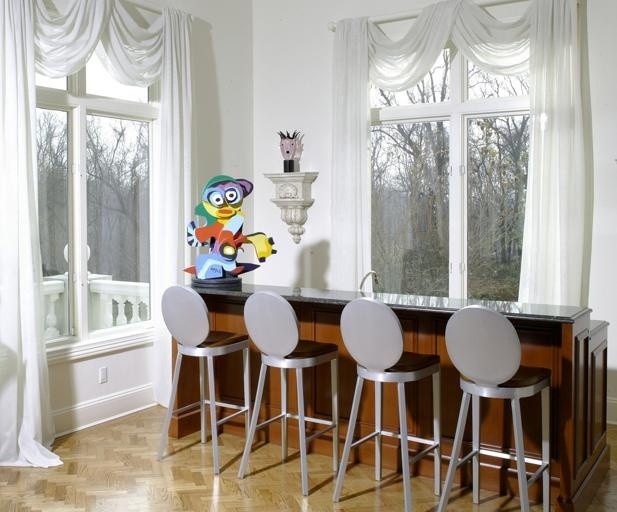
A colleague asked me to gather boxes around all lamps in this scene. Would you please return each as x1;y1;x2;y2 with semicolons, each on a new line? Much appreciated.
276;127;300;172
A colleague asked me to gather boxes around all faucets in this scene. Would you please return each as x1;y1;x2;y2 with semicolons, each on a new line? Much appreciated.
359;271;379;293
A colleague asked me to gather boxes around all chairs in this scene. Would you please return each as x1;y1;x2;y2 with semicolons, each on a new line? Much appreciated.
440;303;554;512
159;284;255;476
237;290;342;499
329;295;444;512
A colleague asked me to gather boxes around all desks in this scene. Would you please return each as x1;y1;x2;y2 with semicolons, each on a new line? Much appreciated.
166;276;612;511
